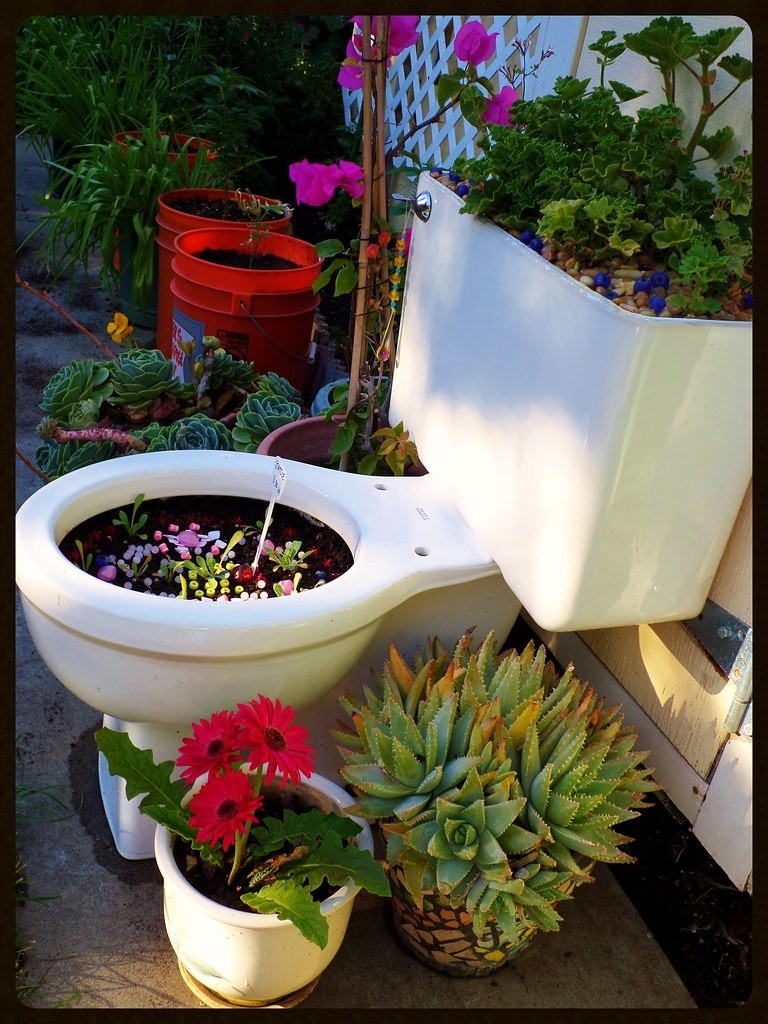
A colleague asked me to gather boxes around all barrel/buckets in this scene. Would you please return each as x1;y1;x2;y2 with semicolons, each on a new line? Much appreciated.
103;130;325;395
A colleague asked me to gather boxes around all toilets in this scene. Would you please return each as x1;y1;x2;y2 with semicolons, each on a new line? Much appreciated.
16;168;751;861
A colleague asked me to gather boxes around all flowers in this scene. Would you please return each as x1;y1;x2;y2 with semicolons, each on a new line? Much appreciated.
200;127;279;220
105;312;140;350
256;15;519;476
94;692;391;952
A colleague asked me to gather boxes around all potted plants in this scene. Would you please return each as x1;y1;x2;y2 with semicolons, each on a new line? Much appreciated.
155;189;294;361
171;188;325;390
389;15;752;632
324;625;664;978
14;128;277;340
16;448;522;860
30;347;304;482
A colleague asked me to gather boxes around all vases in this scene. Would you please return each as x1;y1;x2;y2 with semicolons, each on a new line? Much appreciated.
257;413;429;477
154;763;374;1008
109;130;217;274
